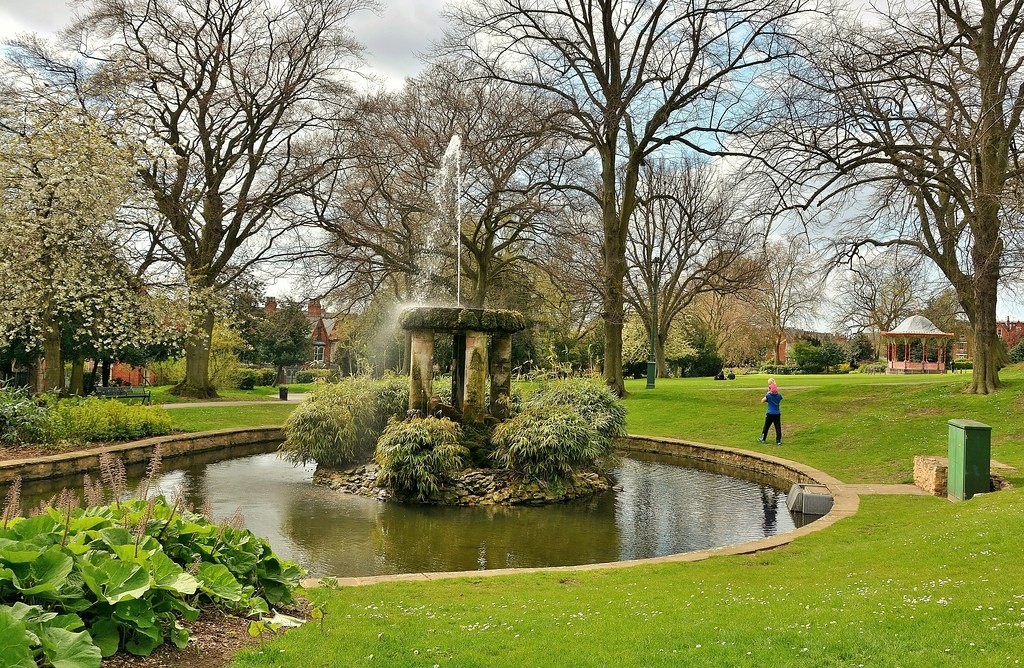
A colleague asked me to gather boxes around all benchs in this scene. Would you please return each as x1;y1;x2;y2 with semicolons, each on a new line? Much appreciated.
95;386;151;406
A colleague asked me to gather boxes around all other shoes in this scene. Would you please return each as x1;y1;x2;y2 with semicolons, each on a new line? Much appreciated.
758;438;765;444
775;441;781;445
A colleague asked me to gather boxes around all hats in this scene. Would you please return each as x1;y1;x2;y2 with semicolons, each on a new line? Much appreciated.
769;384;779;393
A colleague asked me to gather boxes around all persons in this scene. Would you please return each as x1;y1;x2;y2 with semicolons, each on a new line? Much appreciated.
717;371;735;380
758;378;783;445
950;360;956;373
